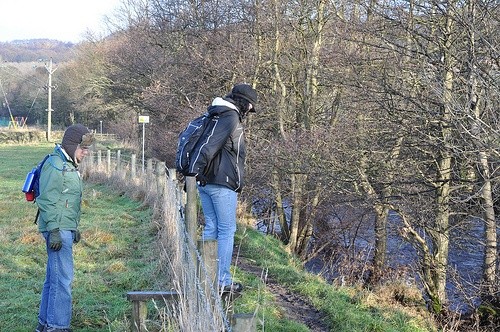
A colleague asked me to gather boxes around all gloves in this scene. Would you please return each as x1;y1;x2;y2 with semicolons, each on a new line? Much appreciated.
192;173;208;187
73;230;81;243
48;228;63;251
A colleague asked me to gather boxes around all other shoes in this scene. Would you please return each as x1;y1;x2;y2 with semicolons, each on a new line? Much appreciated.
35;322;73;332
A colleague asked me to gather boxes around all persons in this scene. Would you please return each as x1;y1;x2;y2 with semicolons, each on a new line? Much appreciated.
35;124;92;332
183;84;259;293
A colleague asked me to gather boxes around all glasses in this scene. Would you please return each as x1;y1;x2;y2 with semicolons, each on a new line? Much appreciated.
80;144;92;149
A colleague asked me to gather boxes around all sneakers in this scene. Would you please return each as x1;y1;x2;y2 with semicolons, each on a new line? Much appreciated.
220;283;242;295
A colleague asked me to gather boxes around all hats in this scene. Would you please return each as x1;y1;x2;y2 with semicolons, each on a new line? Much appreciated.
61;124;95;167
231;84;257;113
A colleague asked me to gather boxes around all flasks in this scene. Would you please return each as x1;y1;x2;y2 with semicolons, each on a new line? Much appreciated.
22;168;38;193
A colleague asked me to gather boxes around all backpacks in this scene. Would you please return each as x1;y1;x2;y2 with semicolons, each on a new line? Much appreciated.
176;107;241;172
23;152;64;201
